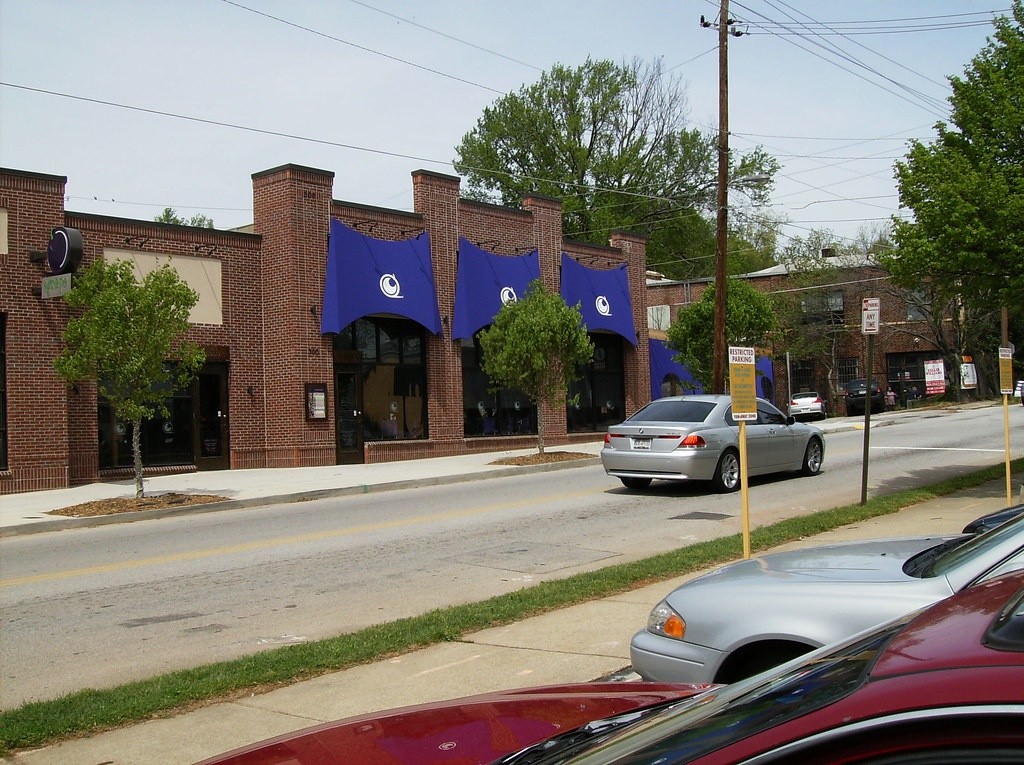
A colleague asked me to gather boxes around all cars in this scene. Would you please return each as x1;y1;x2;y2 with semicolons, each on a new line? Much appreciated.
193;501;1024;765
601;394;827;492
785;393;828;419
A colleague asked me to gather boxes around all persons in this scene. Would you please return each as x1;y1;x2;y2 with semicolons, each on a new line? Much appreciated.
885;387;898;411
906;386;921;408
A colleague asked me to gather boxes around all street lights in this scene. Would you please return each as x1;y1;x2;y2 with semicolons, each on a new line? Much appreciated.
710;172;770;394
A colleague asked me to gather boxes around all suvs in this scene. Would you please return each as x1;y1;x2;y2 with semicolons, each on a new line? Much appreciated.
844;379;888;416
